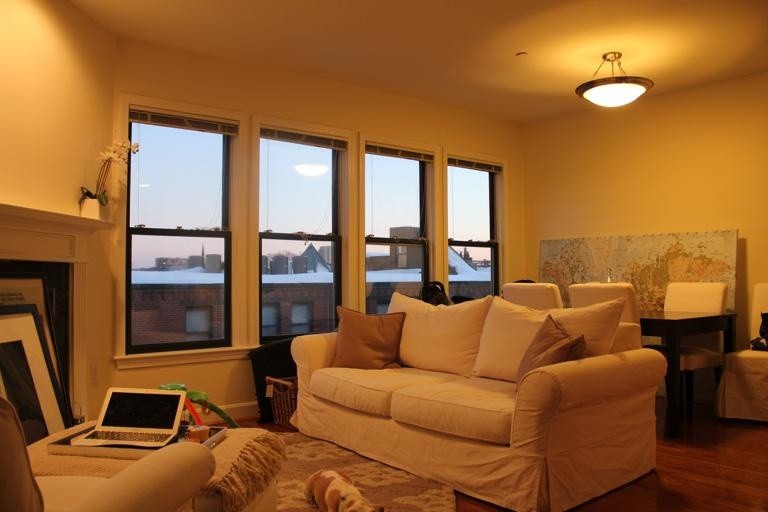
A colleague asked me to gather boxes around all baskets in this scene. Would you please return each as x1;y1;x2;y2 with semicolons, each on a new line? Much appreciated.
265;374;298;433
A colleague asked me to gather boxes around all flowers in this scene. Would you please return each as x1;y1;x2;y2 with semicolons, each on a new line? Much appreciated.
96;138;139;198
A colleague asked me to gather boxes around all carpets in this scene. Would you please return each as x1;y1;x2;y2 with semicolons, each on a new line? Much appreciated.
273;433;457;512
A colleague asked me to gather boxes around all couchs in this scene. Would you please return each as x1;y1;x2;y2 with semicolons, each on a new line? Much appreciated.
291;325;669;511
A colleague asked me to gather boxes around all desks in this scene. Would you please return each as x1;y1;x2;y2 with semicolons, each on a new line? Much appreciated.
25;421;290;512
640;309;737;439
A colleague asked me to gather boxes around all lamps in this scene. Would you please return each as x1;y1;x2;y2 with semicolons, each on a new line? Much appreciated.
575;51;655;108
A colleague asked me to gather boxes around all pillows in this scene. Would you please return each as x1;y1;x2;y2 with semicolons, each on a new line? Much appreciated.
471;296;624;382
333;305;406;368
517;314;586;389
386;292;491;376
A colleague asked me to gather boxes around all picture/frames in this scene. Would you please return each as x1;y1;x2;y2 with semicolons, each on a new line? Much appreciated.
0;272;74;427
0;304;70;447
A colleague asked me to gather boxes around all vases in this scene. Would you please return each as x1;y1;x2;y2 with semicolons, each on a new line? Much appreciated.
80;197;102;216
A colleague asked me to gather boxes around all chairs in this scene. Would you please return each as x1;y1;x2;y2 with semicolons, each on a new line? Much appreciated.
568;282;639;323
0;395;216;512
643;282;728;432
716;282;768;422
503;283;562;309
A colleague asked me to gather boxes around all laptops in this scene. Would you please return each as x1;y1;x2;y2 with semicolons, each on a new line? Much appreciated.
75;386;187;448
